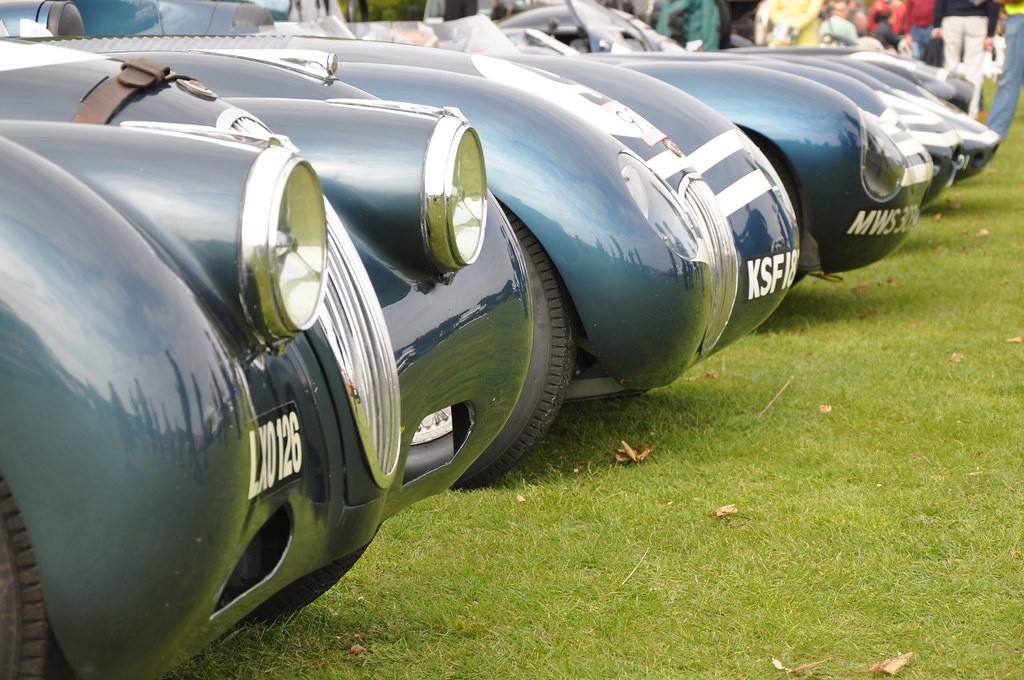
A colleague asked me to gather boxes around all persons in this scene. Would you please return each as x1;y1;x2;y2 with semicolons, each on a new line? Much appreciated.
650;2;1022;165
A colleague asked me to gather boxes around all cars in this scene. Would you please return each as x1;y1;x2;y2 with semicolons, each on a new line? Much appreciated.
0;0;1003;680
